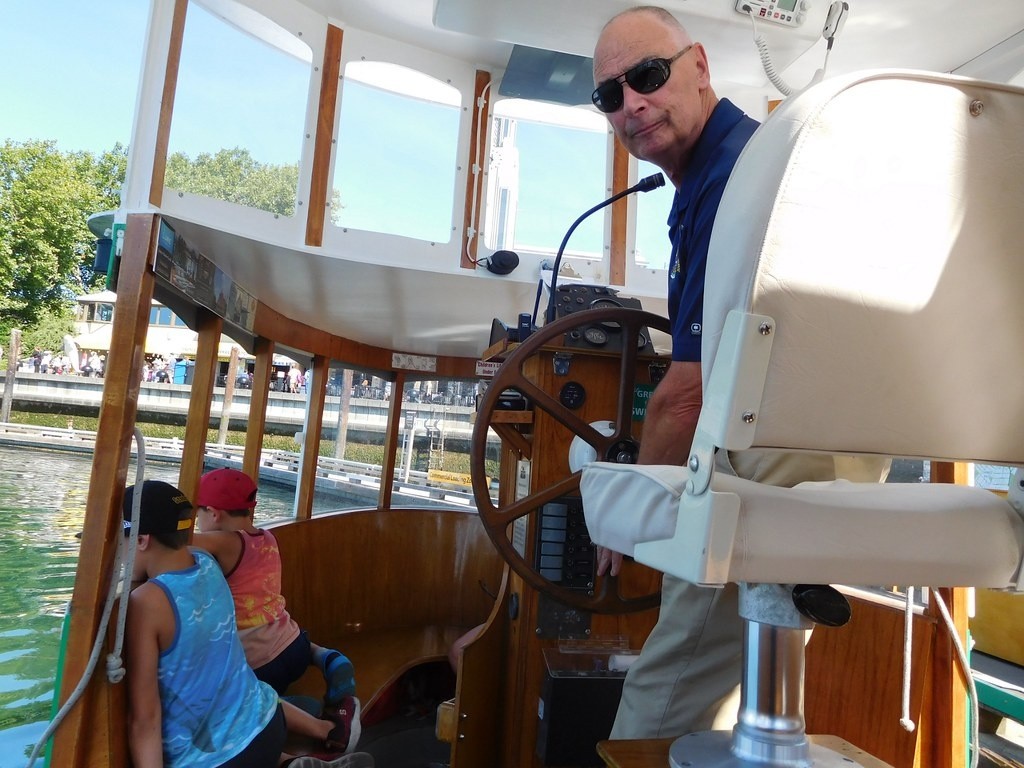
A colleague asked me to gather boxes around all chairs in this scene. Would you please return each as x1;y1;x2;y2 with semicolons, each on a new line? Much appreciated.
581;67;1024;768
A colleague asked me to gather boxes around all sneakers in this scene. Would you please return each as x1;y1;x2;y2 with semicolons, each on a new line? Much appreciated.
280;751;375;768
325;696;361;754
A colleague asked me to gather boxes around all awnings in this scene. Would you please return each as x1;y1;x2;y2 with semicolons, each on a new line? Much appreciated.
78;324;299;367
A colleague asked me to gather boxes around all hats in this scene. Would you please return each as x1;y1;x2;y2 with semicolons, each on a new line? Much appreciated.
75;480;192;539
197;467;258;509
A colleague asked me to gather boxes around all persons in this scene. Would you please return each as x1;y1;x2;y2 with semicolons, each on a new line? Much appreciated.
190;467;355;705
585;6;769;740
31;346;479;408
73;480;343;768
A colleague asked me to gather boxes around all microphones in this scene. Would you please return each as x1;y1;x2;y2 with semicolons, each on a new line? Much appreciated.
546;172;665;326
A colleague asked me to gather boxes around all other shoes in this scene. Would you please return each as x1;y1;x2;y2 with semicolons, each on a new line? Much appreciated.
321;649;356;701
280;696;322;718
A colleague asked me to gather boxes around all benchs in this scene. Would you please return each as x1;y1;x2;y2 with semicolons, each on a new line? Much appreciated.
81;507;504;768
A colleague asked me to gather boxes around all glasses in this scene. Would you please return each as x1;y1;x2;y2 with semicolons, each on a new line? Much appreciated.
591;45;692;113
